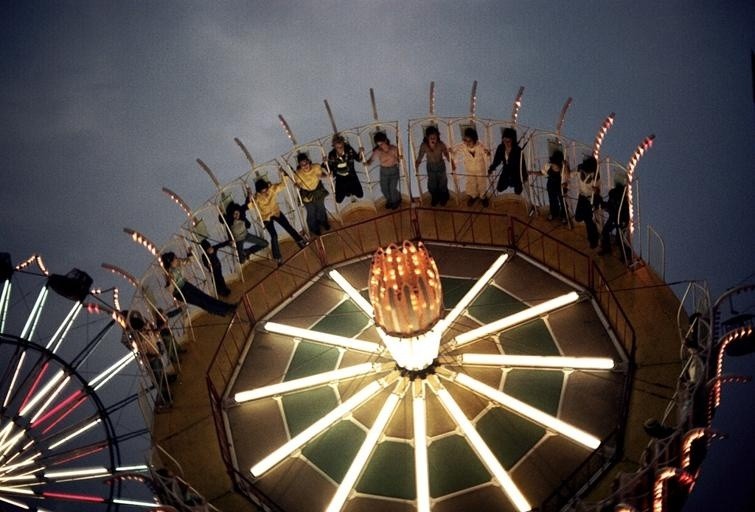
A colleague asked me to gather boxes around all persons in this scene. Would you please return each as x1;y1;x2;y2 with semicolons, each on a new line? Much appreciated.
449;128;492;208
321;136;365;203
527;150;572;225
596;308;709;511
294;153;333;236
593;173;632;264
563;155;603;249
247;166;310;267
487;128;529;195
363;132;403;211
415;126;457;207
119;186;269;404
